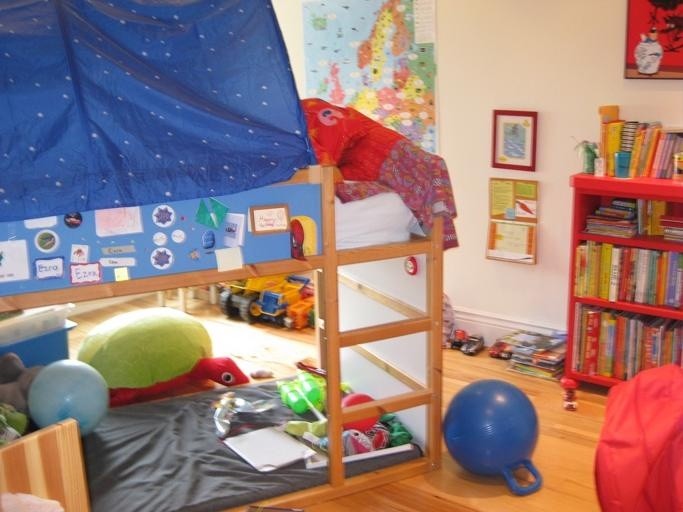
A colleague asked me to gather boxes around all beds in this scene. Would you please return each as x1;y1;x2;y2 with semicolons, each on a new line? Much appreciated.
0;0;444;512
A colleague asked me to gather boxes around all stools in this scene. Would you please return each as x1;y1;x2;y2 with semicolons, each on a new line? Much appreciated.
157;284;220;313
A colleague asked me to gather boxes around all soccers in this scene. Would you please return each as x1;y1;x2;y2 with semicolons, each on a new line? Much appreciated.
29;360;108;436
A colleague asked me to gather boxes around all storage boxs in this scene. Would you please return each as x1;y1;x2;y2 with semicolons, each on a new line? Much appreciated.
0;303;78;379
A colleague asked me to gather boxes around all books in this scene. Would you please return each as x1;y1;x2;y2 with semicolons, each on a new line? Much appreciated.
616;118;683;180
498;328;567;381
573;240;683;311
583;198;683;241
571;302;683;382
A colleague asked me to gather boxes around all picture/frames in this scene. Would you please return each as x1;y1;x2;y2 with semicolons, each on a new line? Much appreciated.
492;110;537;171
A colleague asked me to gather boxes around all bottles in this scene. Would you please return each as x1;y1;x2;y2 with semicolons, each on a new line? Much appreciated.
672;153;683;181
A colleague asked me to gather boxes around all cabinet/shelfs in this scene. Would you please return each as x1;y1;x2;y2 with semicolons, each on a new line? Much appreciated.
560;171;683;410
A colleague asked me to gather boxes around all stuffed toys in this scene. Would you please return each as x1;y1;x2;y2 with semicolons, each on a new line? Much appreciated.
275;360;415;456
0;401;29;449
76;306;250;407
0;352;42;415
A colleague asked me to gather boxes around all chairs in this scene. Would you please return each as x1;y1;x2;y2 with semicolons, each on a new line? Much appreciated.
593;362;682;512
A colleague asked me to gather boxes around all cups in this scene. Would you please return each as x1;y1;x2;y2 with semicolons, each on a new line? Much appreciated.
616;152;630;178
594;158;603;176
